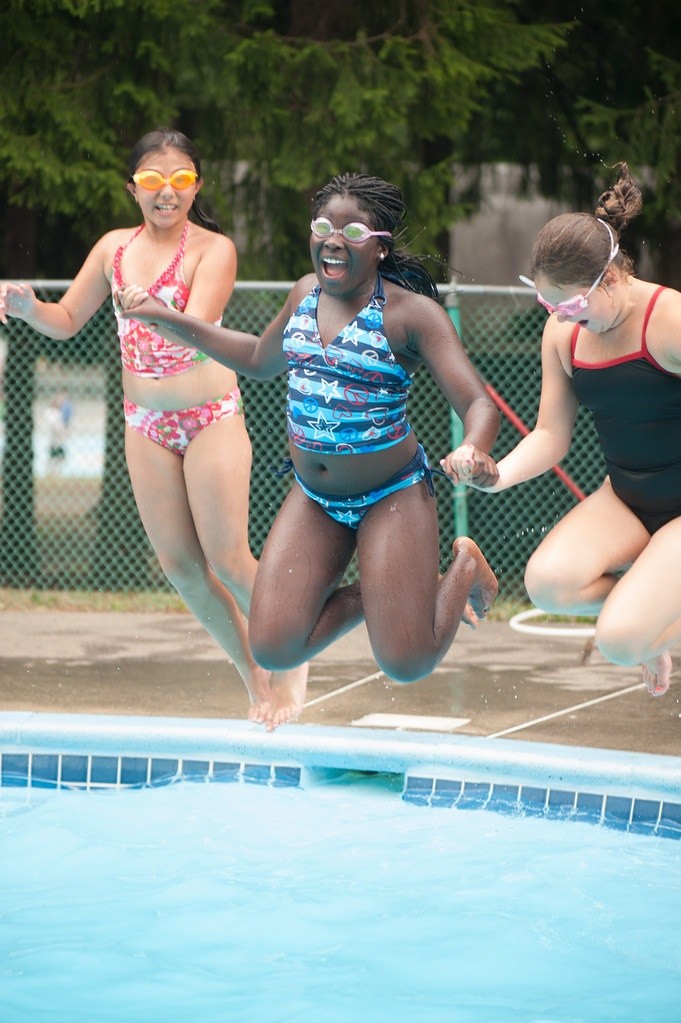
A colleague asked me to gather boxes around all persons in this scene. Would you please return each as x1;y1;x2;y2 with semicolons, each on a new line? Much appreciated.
439;162;681;699
112;173;501;685
0;128;309;731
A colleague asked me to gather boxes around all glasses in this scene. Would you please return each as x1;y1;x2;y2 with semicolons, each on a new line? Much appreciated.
536;218;619;317
310;217;391;243
132;169;197;192
